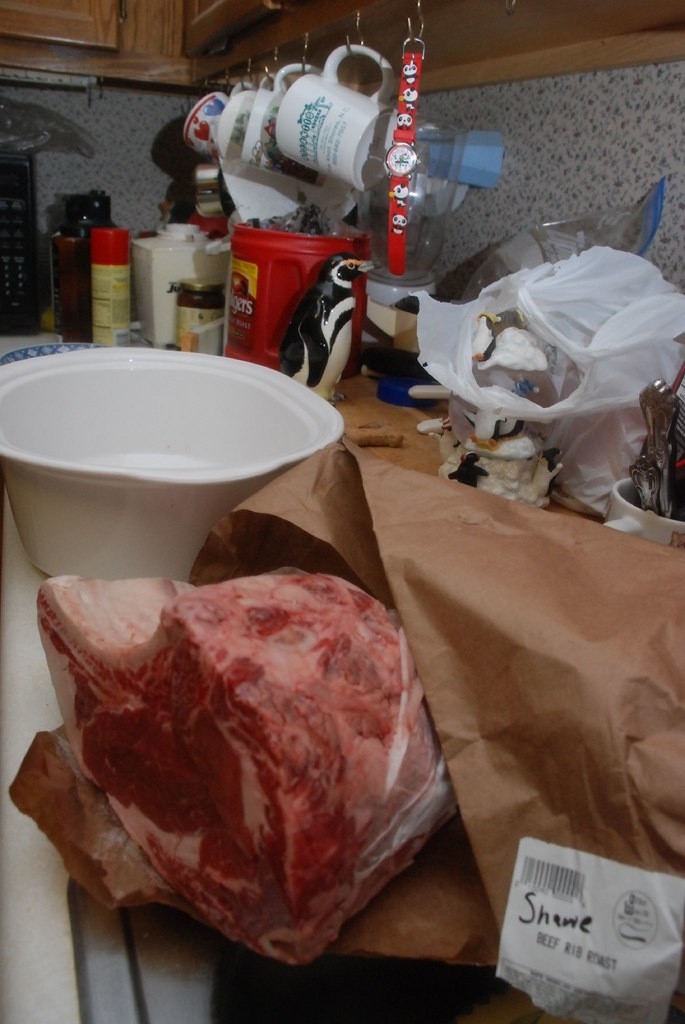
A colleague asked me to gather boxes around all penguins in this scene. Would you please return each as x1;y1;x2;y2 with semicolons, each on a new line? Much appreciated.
277;251;375;407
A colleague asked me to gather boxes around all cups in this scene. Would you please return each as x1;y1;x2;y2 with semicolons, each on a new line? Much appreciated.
276;43;400;190
242;62;330;187
217;81;261;161
602;475;685;549
182;91;231;161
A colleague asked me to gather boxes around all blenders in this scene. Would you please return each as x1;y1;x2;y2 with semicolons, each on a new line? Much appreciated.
357;113;469;376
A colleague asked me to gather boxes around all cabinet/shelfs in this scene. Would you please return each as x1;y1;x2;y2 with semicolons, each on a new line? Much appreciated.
0;0;685;92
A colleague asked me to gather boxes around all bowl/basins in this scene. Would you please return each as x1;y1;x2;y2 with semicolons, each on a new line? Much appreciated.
0;347;346;590
